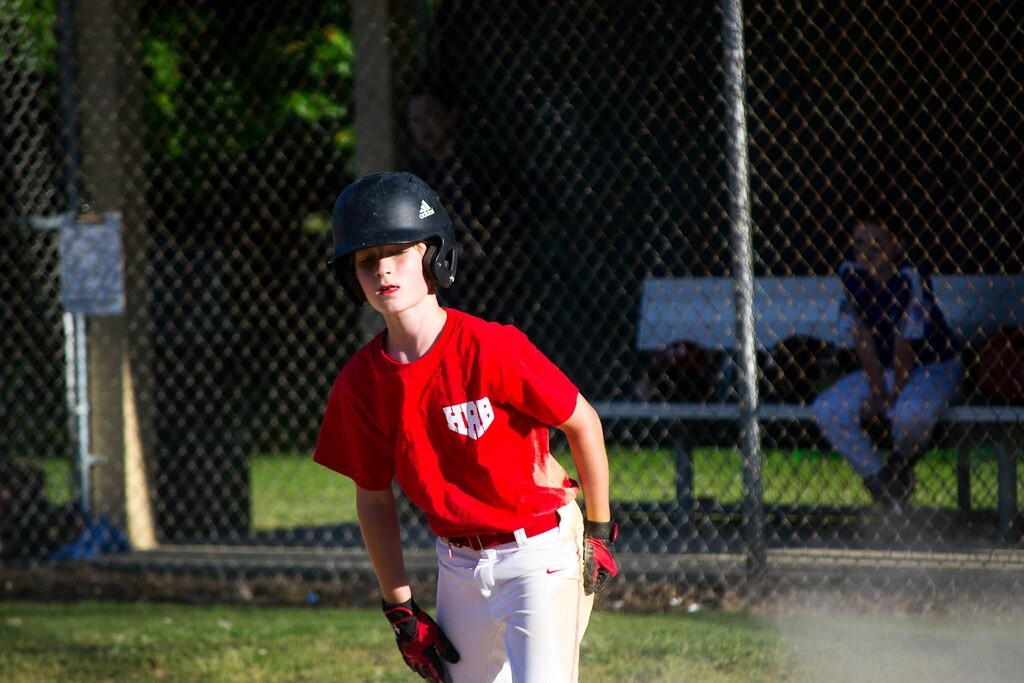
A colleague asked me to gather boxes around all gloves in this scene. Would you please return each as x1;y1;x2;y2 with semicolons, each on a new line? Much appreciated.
583;515;618;596
382;598;460;683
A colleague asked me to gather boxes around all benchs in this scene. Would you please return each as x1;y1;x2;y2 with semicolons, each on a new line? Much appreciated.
587;275;1024;532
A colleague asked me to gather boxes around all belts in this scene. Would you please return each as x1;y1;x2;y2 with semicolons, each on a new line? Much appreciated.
441;512;560;551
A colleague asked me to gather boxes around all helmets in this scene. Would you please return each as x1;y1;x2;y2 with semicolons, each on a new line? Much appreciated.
327;171;458;308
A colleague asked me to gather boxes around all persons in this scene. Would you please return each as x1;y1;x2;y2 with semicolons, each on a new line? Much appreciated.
313;172;617;683
813;220;961;516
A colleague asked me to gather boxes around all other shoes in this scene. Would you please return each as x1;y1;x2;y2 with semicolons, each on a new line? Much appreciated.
863;468;915;509
